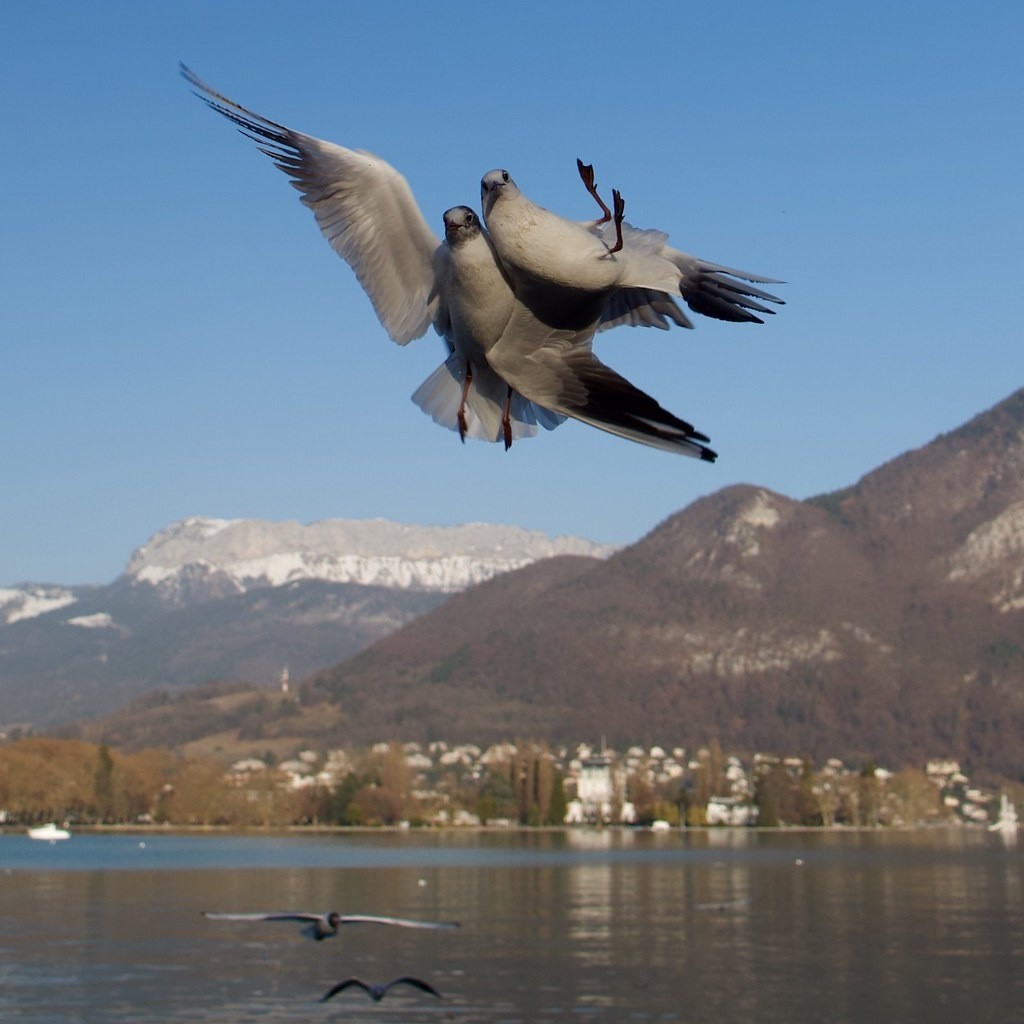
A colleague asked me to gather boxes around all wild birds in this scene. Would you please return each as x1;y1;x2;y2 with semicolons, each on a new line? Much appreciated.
319;976;444;1003
178;59;789;463
199;909;467;943
